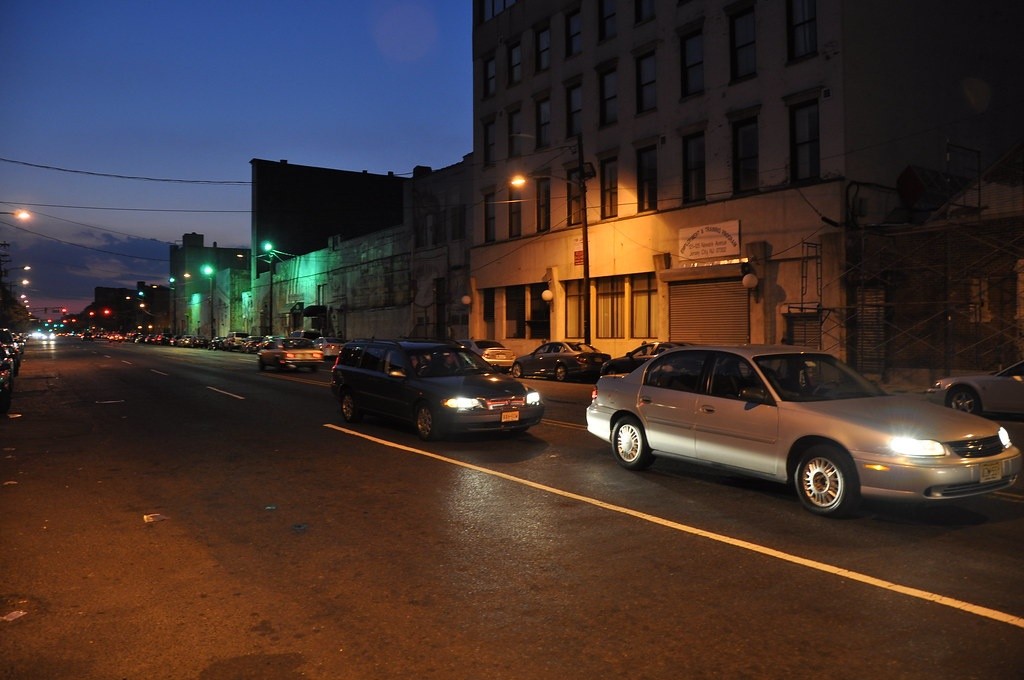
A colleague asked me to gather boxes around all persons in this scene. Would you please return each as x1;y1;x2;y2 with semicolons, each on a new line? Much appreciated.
443;352;457;372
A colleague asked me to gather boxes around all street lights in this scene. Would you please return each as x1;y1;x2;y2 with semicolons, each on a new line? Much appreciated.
200;264;216;341
510;175;591;345
262;242;273;335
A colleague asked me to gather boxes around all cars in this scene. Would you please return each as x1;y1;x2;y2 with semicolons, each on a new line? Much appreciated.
585;345;1022;518
80;326;346;358
455;338;517;374
0;327;31;413
599;340;699;379
256;336;326;373
924;360;1024;416
511;341;611;382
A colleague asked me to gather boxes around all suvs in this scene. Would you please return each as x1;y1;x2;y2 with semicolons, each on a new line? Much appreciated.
329;337;545;444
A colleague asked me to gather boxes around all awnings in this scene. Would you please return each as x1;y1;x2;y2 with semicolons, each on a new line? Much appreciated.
278;302;303;314
301;305;326;317
